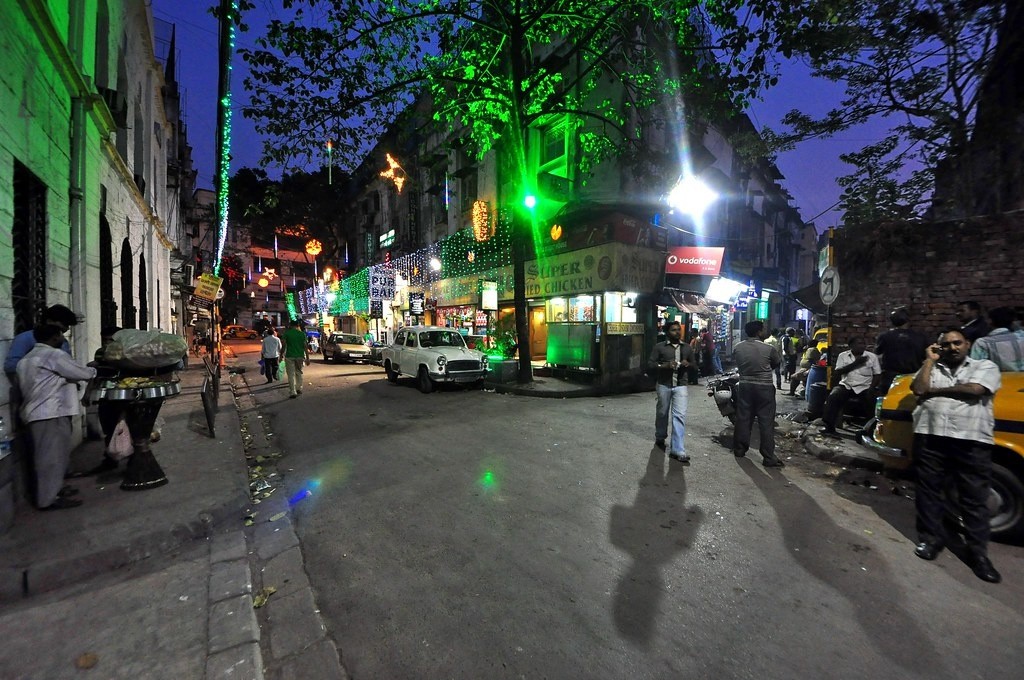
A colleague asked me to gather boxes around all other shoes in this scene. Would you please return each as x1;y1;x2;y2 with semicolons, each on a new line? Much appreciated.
297;390;303;394
655;439;669;450
669;452;690;461
734;451;744;457
265;381;273;384
273;376;277;380
38;487;83;511
819;427;835;433
289;395;297;398
787;391;794;396
763;460;784;466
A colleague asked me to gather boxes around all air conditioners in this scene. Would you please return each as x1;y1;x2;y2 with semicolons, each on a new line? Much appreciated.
97;87;128;126
134;175;146;197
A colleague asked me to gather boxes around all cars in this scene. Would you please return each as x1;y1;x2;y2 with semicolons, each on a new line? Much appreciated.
221;325;258;339
323;333;372;365
381;325;492;393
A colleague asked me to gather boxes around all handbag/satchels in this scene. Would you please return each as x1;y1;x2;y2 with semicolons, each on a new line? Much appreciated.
258;357;266;375
276;361;286;384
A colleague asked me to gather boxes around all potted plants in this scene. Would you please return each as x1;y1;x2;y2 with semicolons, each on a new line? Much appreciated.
475;313;521;384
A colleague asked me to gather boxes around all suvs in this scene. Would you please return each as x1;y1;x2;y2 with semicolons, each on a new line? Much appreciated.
872;369;1024;543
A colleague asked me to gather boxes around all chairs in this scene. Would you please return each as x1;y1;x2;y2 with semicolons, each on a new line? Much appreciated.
337;338;344;343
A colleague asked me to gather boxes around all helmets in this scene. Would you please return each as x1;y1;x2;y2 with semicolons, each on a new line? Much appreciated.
713;386;732;403
888;305;910;323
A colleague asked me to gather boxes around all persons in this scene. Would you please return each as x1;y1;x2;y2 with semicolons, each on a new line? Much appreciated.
645;299;1024;470
260;319;320;398
908;326;1002;584
3;304;122;510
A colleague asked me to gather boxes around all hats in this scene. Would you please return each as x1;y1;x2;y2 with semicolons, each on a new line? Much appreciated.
786;327;795;334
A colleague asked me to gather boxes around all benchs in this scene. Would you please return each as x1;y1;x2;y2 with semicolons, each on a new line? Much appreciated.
828;366;885;422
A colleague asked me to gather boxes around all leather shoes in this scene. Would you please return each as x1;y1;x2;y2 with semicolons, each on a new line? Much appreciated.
915;540;947;561
963;548;1003;583
